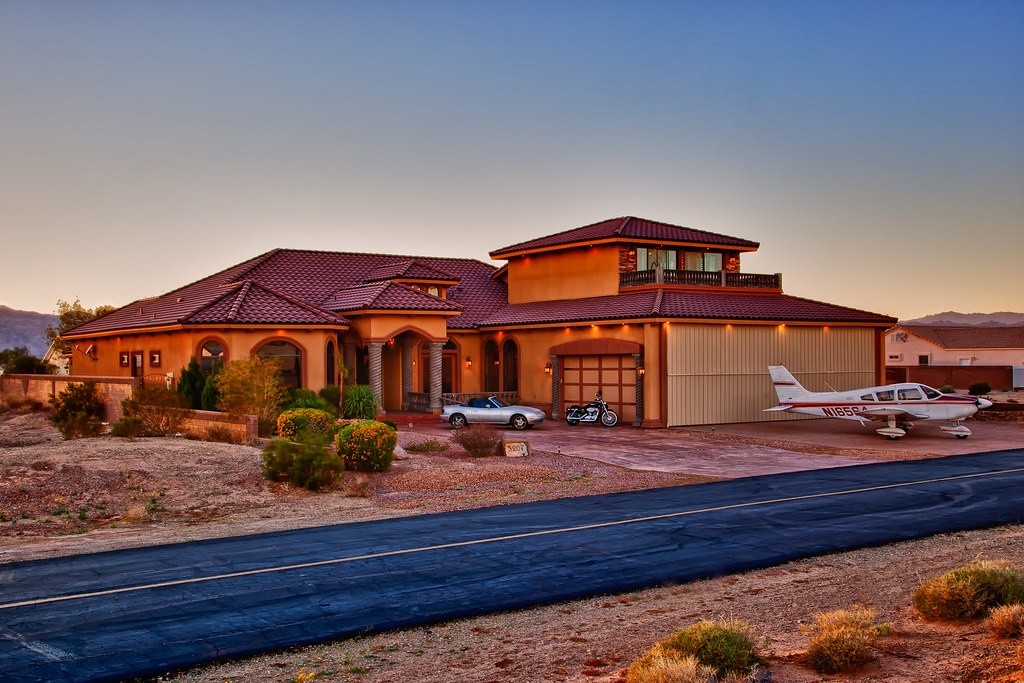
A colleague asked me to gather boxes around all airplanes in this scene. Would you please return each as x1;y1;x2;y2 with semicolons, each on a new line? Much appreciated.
761;363;993;440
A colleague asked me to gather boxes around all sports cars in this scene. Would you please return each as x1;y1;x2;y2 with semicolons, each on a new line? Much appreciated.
439;395;546;429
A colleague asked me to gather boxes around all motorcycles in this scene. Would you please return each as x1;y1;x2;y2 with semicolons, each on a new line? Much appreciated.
565;391;618;427
